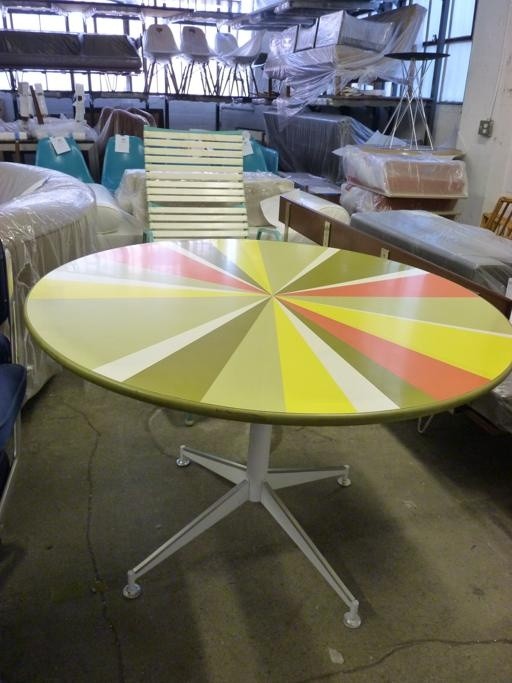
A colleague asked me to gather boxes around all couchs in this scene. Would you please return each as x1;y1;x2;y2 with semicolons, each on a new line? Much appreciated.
2;159;94;413
262;5;427;99
1;30;141;93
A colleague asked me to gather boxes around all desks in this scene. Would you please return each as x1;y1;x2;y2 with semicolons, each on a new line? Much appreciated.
23;236;512;629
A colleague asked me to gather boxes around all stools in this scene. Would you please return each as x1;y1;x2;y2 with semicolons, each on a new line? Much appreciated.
143;23;178;95
380;51;450;157
216;32;255;101
181;25;217;99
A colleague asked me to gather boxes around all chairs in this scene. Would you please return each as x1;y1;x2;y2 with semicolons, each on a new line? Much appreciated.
95;107;114;133
130;107;157;129
139;125;246;242
100;110;146;144
100;136;145;193
1;243;32;519
242;138;267;173
37;138;93;189
